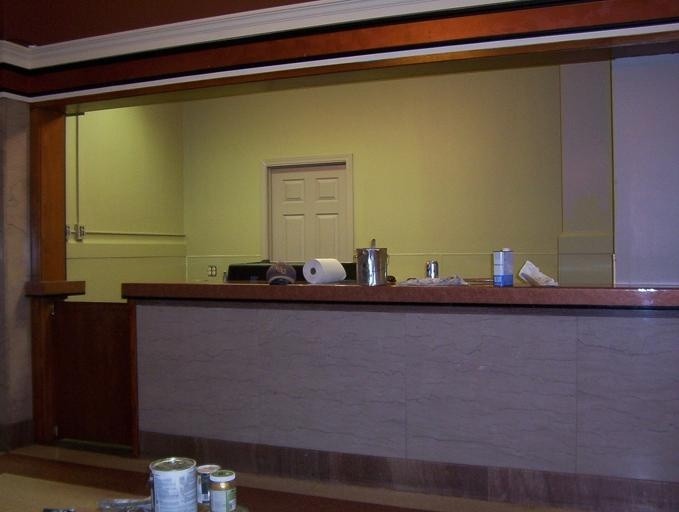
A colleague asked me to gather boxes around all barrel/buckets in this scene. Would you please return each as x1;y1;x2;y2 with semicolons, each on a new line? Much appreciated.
353;248;388;288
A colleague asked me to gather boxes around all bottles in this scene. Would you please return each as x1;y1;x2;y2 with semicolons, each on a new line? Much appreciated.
209;470;237;512
